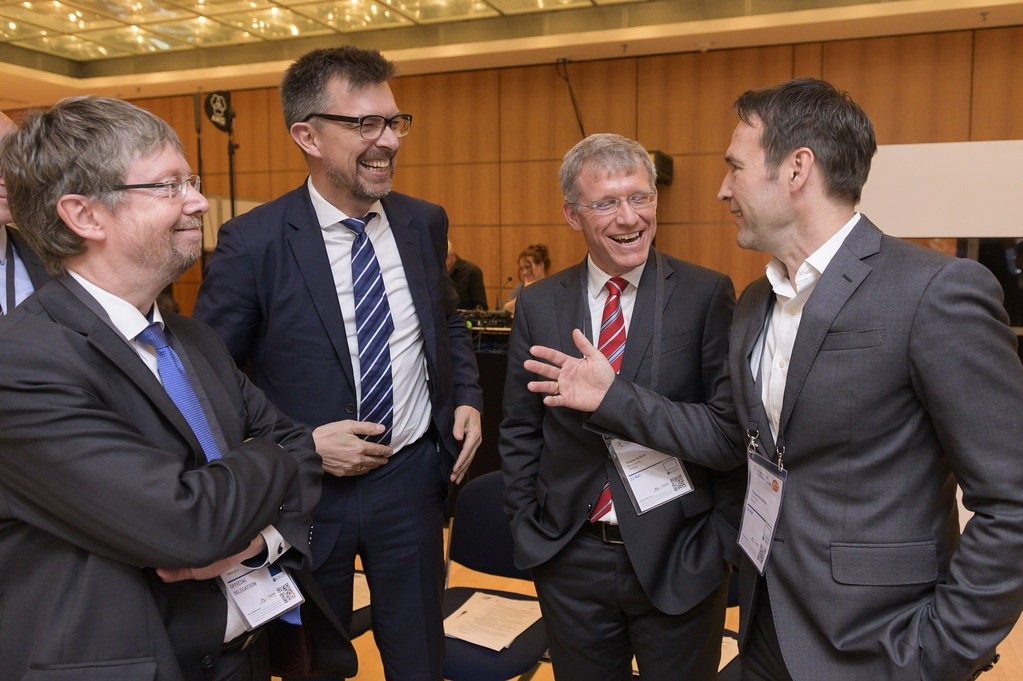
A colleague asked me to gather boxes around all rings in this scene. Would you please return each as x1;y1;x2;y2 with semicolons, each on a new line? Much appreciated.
553;382;559;395
358;465;361;470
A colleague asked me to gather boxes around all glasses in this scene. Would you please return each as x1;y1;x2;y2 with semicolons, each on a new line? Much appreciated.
302;112;413;139
89;175;200;203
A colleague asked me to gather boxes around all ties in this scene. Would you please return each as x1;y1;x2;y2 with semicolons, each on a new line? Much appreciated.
133;322;301;627
340;211;394;446
586;276;627;523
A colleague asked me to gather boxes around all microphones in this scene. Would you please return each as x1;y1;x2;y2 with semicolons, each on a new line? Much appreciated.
494;276;513;311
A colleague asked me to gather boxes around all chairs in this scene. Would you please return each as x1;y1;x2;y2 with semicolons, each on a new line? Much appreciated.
441;468;548;681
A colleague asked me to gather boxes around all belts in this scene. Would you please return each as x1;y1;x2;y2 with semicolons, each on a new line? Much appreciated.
574;520;625;545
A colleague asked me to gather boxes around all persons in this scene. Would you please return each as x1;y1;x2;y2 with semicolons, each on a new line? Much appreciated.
0;107;50;317
504;242;552;319
189;46;484;681
496;133;736;681
0;94;392;681
523;76;1022;681
446;239;490;311
